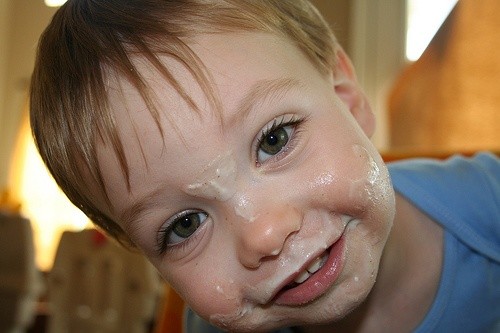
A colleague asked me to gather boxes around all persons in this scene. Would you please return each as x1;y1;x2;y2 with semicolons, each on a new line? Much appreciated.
29;0;499;333
